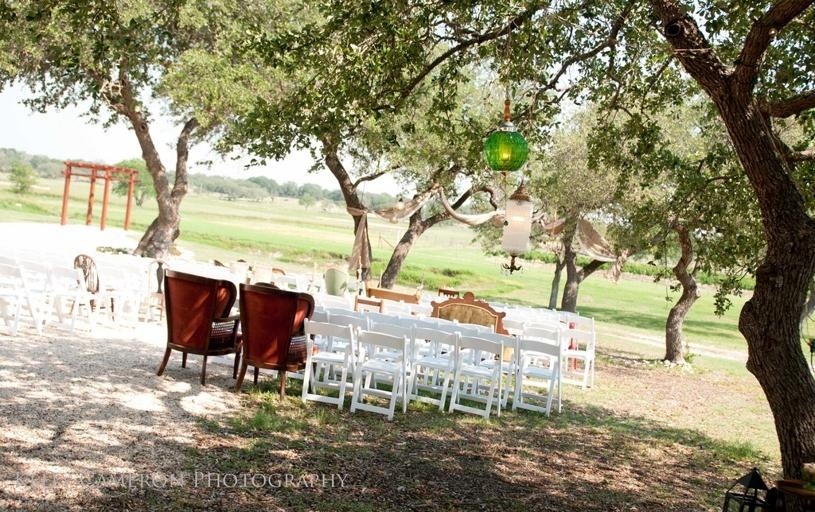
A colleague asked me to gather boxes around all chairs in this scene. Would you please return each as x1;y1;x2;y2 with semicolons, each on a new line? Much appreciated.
156;259;598;423
0;227;163;337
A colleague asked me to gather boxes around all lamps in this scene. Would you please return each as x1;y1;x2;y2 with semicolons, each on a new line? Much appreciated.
484;24;529;174
499;181;536;274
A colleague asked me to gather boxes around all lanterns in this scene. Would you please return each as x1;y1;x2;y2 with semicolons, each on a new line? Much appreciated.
484;132;529;172
501;198;534;254
